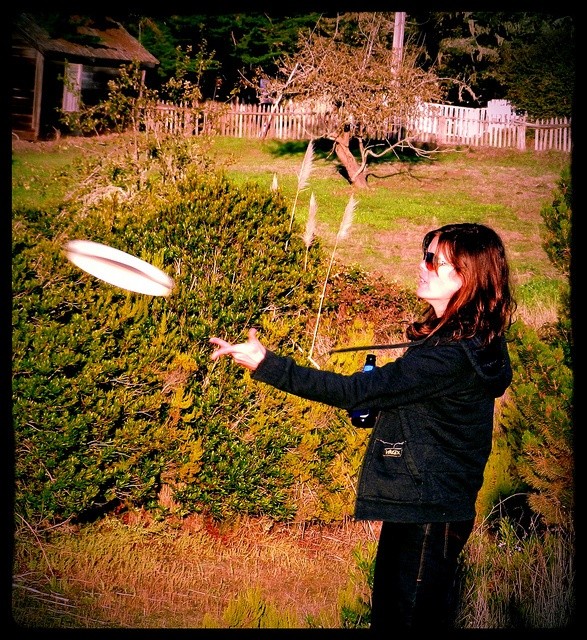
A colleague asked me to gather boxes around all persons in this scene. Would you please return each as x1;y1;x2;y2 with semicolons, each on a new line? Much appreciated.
206;222;514;630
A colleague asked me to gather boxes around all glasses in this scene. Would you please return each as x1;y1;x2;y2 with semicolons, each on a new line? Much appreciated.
422;251;454;273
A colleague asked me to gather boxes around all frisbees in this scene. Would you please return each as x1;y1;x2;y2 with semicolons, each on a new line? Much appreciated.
63;239;175;297
